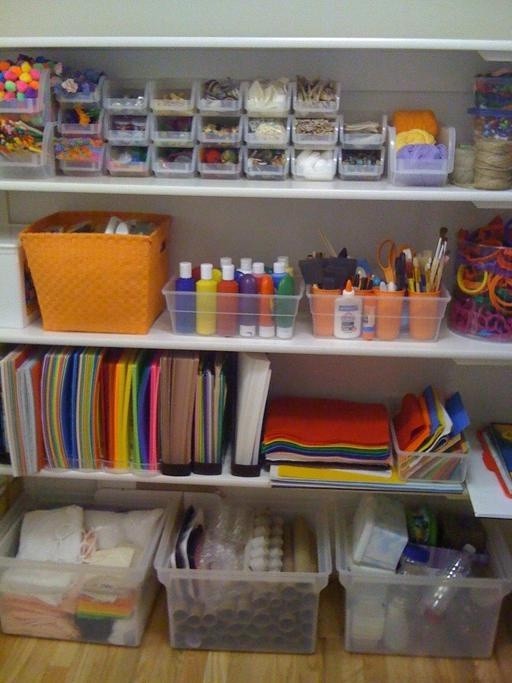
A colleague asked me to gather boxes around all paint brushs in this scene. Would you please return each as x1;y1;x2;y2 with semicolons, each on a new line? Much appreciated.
412;227;450;293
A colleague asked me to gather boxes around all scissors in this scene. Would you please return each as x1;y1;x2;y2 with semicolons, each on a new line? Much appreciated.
376;239;416;284
378;280;400;291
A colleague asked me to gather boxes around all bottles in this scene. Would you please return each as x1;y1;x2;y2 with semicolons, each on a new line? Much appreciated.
335;280;363;337
398;545;430;575
172;252;295;339
422;544;477;619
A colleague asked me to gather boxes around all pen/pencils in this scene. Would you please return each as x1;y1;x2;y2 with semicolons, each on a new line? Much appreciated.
353;270;375;291
380;248;415;293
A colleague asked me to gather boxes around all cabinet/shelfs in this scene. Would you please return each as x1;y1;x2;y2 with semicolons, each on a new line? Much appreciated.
0;35;511;501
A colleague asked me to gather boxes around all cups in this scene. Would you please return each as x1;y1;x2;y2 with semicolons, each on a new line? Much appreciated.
311;284;440;341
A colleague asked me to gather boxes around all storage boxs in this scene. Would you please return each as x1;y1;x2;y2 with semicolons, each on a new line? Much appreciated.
160;269;306;341
0;223;41;330
306;277;452;341
151;488;335;656
0;488;187;649
325;488;511;663
383;394;471;483
